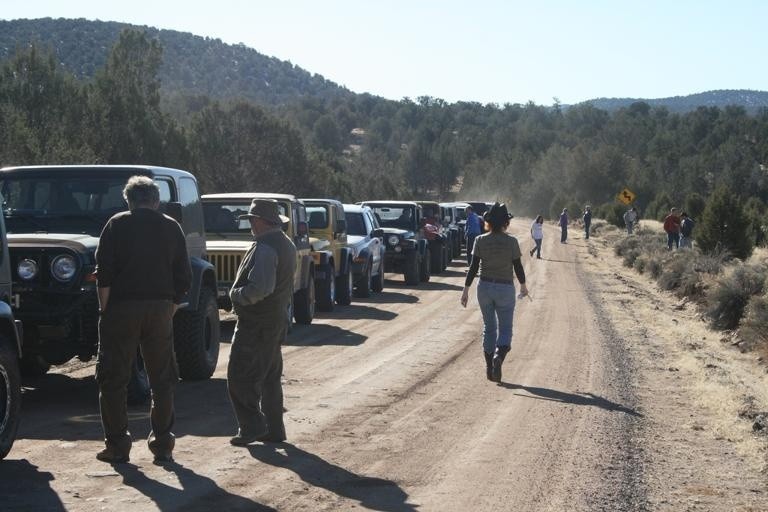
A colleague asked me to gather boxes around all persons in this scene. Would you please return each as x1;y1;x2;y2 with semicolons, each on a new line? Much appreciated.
583;205;592;239
678;212;694;250
663;207;680;249
92;175;193;463
459;202;528;382
622;207;637;235
225;198;297;446
559;208;569;244
464;205;481;276
529;214;544;259
402;208;410;220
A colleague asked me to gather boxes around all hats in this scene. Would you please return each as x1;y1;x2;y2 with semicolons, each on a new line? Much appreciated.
483;201;513;224
236;197;290;225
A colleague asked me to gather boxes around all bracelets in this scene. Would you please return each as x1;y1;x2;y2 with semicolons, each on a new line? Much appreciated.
96;308;105;315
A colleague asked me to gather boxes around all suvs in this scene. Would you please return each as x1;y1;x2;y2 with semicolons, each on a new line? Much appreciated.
1;163;224;406
337;201;386;299
297;197;355;314
1;195;27;464
360;198;434;286
193;188;320;332
419;197;502;291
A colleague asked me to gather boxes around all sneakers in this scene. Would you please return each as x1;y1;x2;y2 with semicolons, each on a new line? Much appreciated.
153;451;172;462
530;250;534;257
256;431;286;443
230;422;270;446
561;241;568;244
536;256;542;260
96;448;130;463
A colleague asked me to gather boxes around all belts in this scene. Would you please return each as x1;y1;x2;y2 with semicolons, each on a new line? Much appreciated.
480;275;515;286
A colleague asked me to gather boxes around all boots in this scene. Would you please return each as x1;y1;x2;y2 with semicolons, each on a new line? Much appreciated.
484;345;511;382
